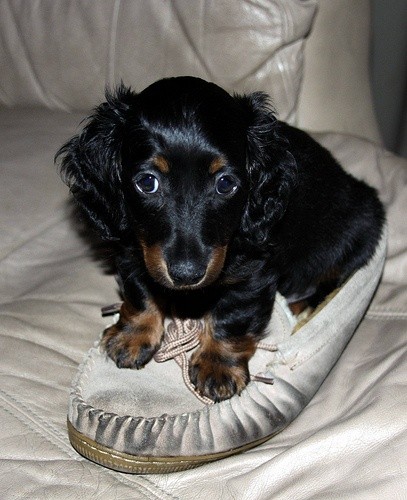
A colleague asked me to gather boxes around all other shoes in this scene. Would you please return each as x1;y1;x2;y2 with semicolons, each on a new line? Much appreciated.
69;235;381;473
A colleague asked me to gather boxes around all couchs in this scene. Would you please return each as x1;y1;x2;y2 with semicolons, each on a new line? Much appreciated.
0;0;407;498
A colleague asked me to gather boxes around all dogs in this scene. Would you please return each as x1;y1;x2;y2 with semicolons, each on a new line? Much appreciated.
53;75;388;405
